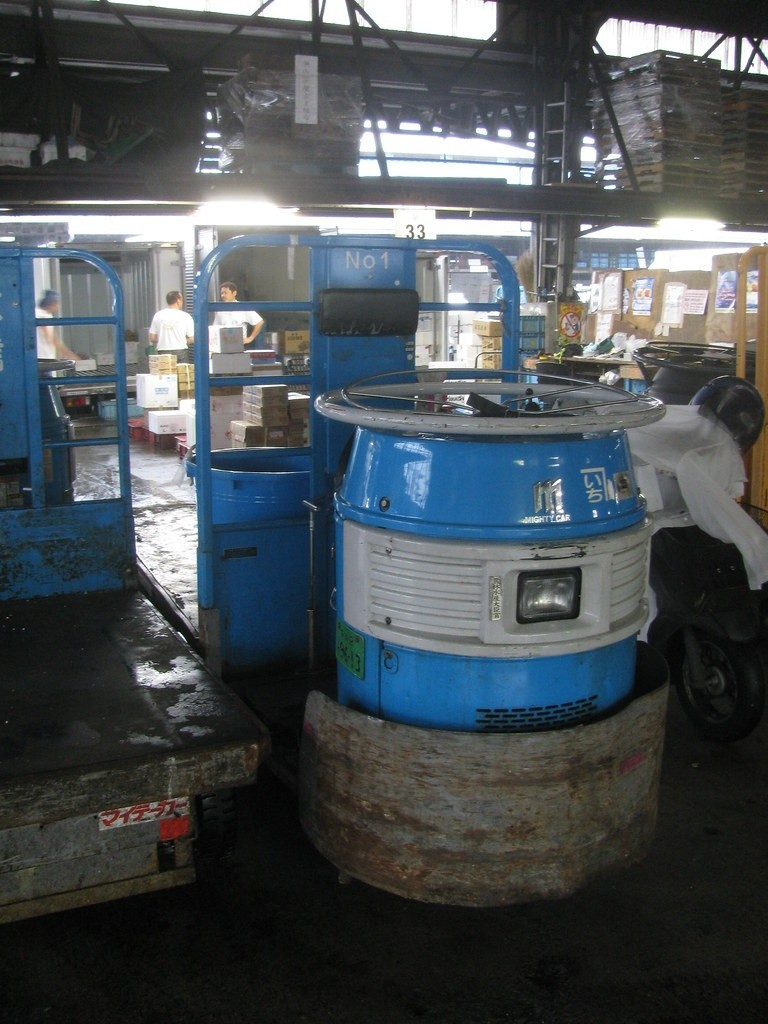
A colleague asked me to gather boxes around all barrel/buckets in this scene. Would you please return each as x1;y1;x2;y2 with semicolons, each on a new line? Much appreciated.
184;446;313;660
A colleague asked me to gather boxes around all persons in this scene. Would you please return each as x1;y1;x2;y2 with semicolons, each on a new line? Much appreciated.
35;290;81;377
213;281;264;349
149;291;194;363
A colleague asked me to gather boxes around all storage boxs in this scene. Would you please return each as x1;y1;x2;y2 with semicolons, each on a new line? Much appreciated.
74;325;309;458
590;50;768;203
470;313;544;382
217;50;365;176
